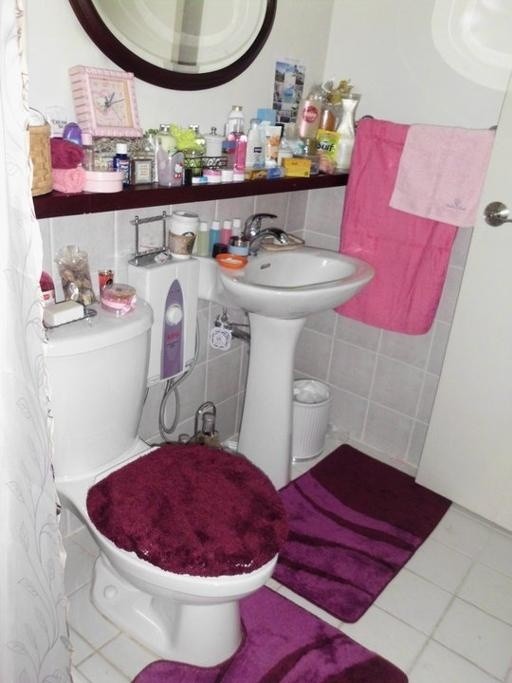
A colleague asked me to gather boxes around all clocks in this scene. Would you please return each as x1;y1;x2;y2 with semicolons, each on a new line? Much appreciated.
69;66;143;140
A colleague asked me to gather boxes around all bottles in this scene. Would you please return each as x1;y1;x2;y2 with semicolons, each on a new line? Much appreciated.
198;218;241;256
226;105;244;136
113;142;133;186
332;97;358;170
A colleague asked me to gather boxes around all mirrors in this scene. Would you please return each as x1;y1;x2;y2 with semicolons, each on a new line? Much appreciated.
71;0;282;95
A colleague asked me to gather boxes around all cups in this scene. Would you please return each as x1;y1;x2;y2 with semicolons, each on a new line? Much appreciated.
97;268;114;297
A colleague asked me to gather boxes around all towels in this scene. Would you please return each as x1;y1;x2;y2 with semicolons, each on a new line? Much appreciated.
328;117;497;336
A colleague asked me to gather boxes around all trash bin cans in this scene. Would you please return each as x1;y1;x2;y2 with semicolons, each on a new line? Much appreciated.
292;379;333;462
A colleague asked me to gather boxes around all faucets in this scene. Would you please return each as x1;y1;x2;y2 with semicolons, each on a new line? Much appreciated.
243;212;292;254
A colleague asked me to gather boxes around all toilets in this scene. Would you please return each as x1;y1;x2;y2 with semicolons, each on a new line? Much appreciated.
39;296;291;668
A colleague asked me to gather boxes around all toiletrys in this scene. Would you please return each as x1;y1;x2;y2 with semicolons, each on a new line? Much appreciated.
196;218;242;257
63;98;359;187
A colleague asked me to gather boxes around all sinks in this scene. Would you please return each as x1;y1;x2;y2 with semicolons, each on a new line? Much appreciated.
217;246;375;319
